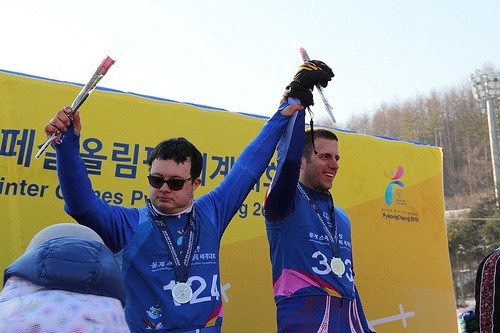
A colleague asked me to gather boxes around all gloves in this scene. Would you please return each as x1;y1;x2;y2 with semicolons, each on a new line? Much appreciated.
284;60;335;108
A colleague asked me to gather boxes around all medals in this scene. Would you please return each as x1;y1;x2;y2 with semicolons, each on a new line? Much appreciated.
330;258;345;276
172;283;192;303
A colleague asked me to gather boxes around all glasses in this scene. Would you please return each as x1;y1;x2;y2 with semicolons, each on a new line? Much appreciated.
147;173;192;191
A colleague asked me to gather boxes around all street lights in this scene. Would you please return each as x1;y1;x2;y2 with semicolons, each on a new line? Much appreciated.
470;66;500;209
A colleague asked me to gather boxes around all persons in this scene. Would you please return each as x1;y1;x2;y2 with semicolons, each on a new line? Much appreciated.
262;59;373;332
45;88;303;332
0;223;131;332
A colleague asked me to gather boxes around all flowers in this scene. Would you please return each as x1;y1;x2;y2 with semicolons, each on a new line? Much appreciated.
300;46;337;124
34;55;116;159
